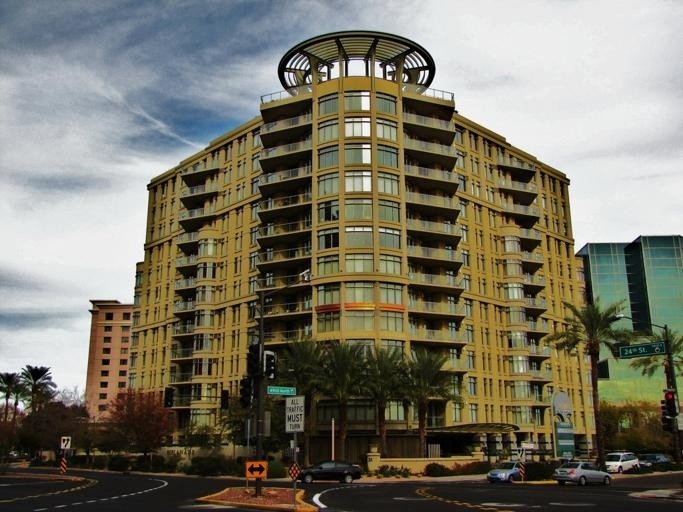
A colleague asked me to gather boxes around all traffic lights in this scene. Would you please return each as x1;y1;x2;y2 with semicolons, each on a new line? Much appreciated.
664;389;679;416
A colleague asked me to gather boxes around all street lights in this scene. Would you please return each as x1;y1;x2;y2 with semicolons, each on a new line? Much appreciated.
255;274;311;497
616;314;677;389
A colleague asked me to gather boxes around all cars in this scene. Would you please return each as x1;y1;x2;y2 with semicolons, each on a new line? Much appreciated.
487;461;522;482
298;461;362;483
605;452;667;473
553;462;611;486
